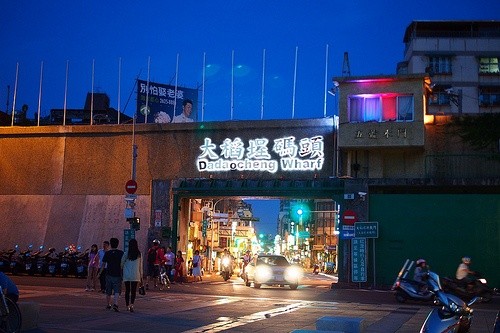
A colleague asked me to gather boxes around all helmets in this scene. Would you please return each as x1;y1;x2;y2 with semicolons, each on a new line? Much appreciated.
153;239;160;244
416;257;426;265
461;255;471;264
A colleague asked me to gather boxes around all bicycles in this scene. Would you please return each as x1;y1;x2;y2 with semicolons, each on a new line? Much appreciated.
154;260;171;291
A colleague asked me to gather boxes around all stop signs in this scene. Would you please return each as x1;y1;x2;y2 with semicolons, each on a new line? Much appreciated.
341;209;358;226
124;178;137;194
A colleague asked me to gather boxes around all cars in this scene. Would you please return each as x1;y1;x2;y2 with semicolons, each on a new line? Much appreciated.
242;254;300;290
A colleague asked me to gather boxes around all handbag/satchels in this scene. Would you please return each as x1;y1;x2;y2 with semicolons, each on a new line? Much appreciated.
139;282;146;295
193;262;198;267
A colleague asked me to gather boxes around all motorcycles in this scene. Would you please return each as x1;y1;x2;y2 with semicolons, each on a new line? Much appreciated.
389;250;499;333
0;241;89;278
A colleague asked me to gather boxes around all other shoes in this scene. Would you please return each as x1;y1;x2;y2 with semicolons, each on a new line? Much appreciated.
104;304;135;313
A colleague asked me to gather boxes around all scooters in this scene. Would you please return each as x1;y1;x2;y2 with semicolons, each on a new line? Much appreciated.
219;257;235;281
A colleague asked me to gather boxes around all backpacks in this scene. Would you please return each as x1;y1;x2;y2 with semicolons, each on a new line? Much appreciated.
147;247;159;262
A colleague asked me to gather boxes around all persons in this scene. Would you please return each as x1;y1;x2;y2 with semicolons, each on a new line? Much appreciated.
120;239;143;312
92;241;109;293
142;239;336;292
85;244;98;292
455;256;479;292
413;259;430;295
171;97;196;124
98;238;124;312
0;272;18;304
